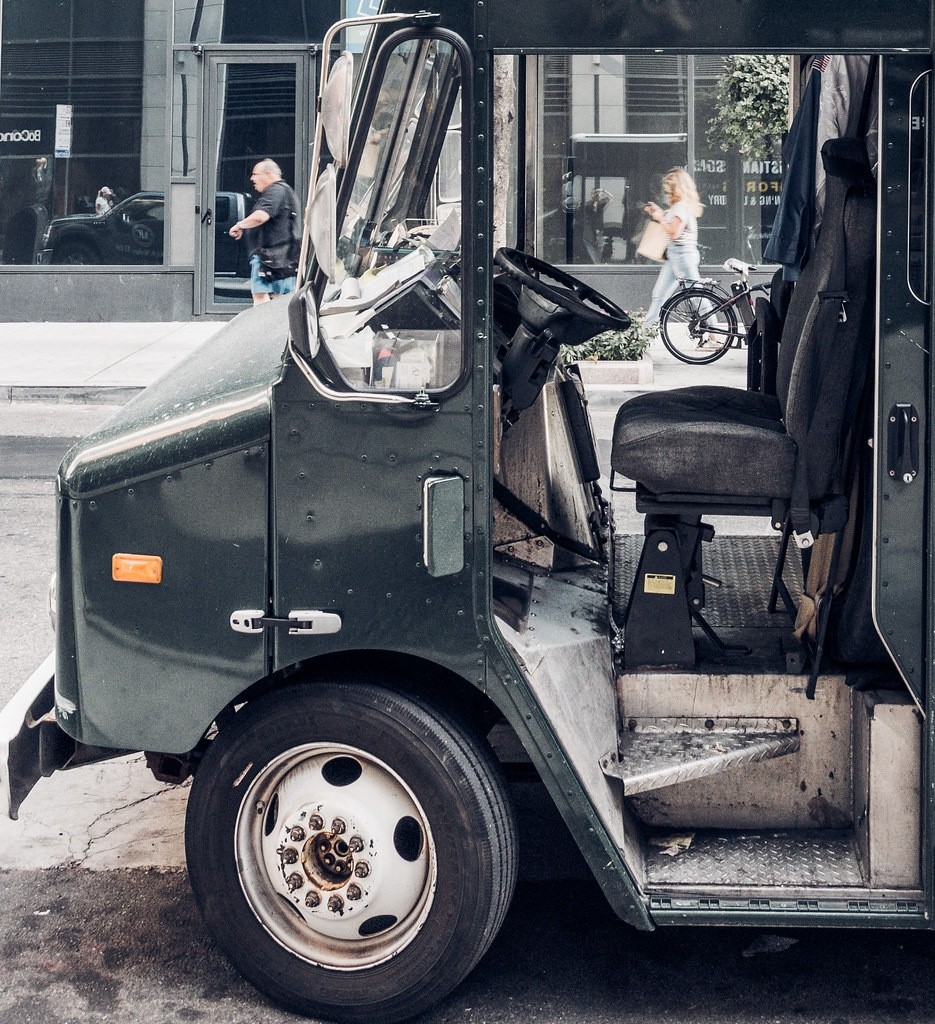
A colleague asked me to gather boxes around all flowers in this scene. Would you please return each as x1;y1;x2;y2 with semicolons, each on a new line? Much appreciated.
557;305;665;362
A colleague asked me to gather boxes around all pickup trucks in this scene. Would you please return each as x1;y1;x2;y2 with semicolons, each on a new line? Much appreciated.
35;187;253;280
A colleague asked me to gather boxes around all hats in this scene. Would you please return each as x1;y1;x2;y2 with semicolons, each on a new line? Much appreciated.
101;186;111;194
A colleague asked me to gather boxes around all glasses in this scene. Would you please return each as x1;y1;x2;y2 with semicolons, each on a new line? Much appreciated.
663;191;673;195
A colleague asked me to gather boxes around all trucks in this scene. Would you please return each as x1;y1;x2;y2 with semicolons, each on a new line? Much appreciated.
2;0;934;1024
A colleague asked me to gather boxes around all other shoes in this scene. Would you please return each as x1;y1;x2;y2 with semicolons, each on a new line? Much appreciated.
709;332;720;345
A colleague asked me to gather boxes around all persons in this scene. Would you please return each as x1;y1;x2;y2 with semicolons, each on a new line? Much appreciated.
228;157;300;307
639;167;723;351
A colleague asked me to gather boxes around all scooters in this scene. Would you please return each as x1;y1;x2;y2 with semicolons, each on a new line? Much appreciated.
658;258;774;366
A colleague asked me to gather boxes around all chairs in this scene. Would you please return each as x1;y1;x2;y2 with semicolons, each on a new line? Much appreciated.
607;135;877;670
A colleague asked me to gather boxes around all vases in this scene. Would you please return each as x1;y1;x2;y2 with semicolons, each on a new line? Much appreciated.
573;351;654;386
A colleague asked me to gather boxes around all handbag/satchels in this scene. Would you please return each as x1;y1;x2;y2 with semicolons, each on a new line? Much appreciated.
636;221;670;261
257;240;300;285
